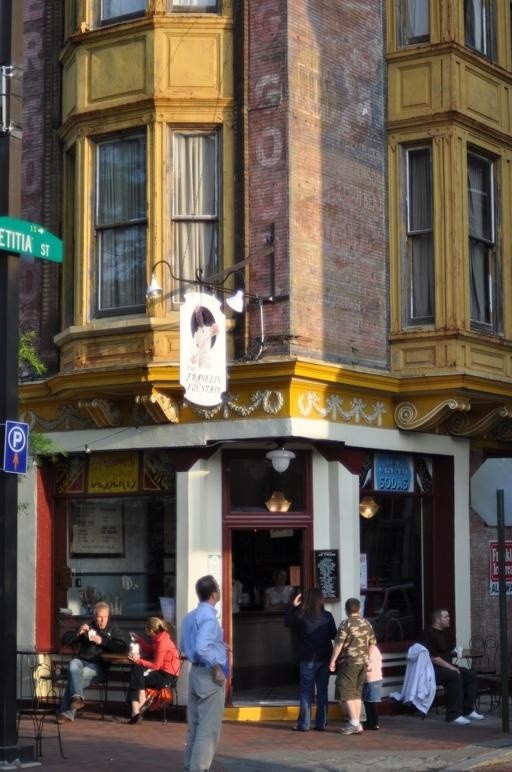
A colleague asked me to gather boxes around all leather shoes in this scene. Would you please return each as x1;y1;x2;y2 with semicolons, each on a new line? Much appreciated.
360;720;379;730
127;714;143;725
139;701;150;714
291;724;310;731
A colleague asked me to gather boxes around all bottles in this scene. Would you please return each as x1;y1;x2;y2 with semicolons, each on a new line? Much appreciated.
456;647;462;658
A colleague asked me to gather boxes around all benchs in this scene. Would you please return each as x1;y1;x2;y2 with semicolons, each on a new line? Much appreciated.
39;659;181;725
378;654;462;718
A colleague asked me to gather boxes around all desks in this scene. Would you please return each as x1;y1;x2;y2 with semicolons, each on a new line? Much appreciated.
450;647;484;712
15;650;56;730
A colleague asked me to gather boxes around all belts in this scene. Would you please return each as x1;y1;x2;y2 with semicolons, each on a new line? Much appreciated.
191;662;206;667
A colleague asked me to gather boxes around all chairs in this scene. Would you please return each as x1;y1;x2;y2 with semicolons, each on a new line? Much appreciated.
10;647;64;760
466;634;499;712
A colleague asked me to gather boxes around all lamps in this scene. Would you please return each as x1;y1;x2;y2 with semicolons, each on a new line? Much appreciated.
359;495;382;519
145;260;273;312
265;484;292;511
265;441;296;473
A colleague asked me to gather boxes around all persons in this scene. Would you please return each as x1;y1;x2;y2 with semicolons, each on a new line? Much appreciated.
126;618;180;723
265;570;296;608
178;575;229;772
420;608;485;725
328;598;378;736
57;602;128;724
286;590;340;731
363;639;387;729
192;306;219;375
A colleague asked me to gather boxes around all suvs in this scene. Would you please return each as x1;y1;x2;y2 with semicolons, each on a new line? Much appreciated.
358;580;420;643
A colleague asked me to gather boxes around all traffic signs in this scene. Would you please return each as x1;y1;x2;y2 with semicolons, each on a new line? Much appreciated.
0;215;65;265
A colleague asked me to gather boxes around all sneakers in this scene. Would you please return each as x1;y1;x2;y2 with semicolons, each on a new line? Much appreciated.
451;716;471;725
466;711;485;721
69;697;83;710
340;723;364;735
57;714;72;725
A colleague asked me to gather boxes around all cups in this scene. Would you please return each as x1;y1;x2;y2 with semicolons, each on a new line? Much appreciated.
111;597;123;615
89;630;96;641
129;643;139;655
